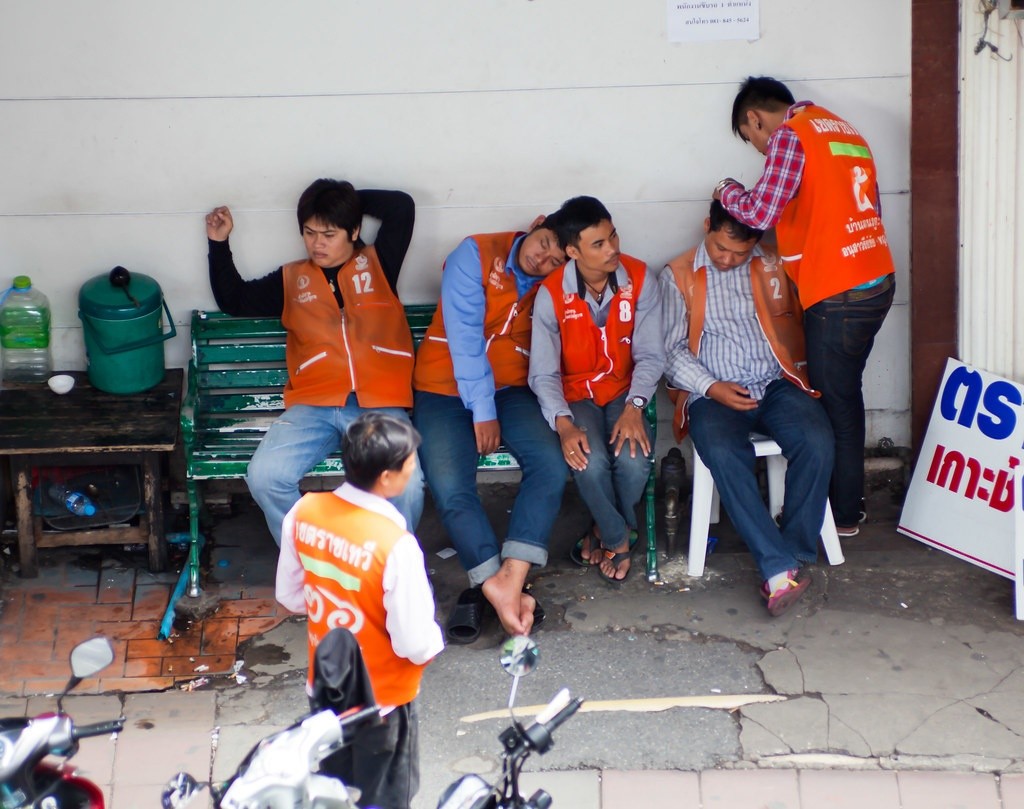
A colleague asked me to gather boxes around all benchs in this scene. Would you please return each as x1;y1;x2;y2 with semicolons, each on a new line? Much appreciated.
180;304;659;597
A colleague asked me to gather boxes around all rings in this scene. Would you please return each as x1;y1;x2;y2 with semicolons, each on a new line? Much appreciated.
570;451;574;455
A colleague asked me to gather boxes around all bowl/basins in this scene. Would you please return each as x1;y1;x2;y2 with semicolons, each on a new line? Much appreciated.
48;374;74;395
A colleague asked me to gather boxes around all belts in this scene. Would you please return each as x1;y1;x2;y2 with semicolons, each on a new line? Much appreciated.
821;272;896;302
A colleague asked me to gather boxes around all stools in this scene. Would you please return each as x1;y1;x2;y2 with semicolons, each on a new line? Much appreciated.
687;433;844;579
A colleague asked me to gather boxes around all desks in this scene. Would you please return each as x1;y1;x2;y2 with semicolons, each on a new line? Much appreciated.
0;368;185;579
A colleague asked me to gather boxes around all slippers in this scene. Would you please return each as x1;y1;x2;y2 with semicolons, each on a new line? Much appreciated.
570;526;604;567
835;511;867;536
760;562;813;617
598;529;640;584
447;589;482;644
521;586;546;633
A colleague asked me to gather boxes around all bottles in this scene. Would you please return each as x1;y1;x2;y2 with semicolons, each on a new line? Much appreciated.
43;482;97;517
0;276;52;383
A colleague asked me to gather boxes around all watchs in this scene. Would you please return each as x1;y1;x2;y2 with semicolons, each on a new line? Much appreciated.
630;396;646;409
716;180;735;195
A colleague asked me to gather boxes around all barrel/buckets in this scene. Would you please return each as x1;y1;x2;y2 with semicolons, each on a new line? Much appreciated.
76;266;177;395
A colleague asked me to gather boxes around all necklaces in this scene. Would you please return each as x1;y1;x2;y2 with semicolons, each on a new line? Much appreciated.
584;277;608;303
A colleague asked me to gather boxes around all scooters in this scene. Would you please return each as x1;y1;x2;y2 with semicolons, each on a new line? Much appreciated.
0;637;127;809
436;636;584;809
162;629;392;809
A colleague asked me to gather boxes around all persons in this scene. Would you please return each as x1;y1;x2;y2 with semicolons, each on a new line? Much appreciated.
657;198;834;616
206;178;426;622
712;77;896;537
528;196;668;581
276;412;445;809
413;212;567;638
